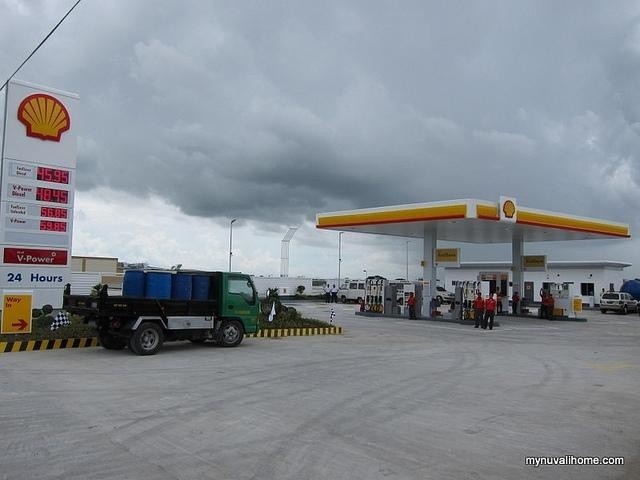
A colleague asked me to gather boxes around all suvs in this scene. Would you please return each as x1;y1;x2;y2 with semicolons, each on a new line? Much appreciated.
599;290;638;315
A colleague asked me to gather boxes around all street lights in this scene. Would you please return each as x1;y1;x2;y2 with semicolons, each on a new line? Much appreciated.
407;240;411;281
339;232;344;288
228;219;236;272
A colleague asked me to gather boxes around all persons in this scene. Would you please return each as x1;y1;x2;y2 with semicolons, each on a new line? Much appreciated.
512;292;519;314
496;286;503;314
483;292;496;330
407;291;416;318
331;284;339;303
547;293;555;317
323;284;332;303
473;292;484;329
539;288;546;319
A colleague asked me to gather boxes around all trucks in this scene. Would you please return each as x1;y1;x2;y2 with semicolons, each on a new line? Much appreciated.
336;280;366;304
62;269;260;356
390;281;413;305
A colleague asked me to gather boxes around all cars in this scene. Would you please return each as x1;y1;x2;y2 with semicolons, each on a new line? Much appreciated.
434;284;455;305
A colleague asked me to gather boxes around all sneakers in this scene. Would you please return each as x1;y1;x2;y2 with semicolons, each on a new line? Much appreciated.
474;325;492;330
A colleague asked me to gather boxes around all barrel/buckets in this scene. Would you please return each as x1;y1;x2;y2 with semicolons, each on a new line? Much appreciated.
122;268;212;301
